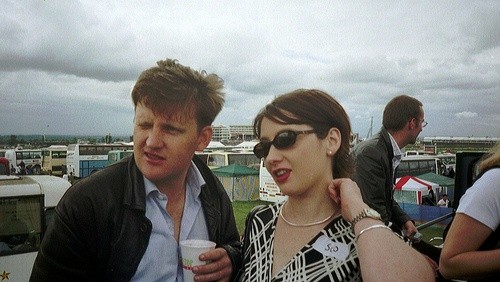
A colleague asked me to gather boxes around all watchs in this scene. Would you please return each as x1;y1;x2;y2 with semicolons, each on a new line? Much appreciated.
350;208;381;234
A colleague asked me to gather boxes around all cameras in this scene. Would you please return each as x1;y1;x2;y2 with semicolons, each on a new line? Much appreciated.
409;232;423;241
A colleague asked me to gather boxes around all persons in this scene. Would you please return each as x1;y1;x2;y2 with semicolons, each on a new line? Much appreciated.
438;143;500;282
437;194;449;207
236;89;438;282
61;164;73;176
29;59;241;282
429;189;434;202
348;95;428;237
448;166;455;178
19;162;30;175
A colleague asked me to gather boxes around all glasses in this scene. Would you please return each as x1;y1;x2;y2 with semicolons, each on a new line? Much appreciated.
416;118;429;127
252;128;320;159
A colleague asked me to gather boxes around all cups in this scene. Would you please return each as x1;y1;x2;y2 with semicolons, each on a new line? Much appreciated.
179;240;216;282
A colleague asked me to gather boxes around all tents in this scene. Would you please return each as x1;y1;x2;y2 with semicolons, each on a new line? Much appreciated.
394;172;455;206
210;163;259;201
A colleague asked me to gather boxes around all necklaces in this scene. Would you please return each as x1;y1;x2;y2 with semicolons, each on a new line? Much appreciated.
280;200;340;227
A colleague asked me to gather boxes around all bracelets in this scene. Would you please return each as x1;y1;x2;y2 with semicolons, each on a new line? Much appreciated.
355;225;391;241
